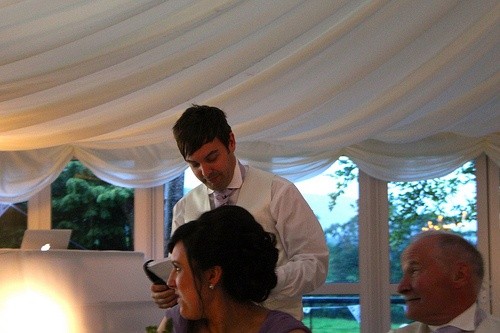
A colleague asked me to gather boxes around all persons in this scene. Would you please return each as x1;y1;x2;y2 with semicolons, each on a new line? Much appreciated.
387;231;500;333
156;205;312;333
150;105;329;323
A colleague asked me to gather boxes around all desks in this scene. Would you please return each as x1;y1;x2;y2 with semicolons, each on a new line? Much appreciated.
1;248;152;333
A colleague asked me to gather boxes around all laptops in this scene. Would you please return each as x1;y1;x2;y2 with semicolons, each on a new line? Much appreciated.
21;229;72;250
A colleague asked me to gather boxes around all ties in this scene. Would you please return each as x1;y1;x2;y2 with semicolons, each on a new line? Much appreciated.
213;188;234;208
